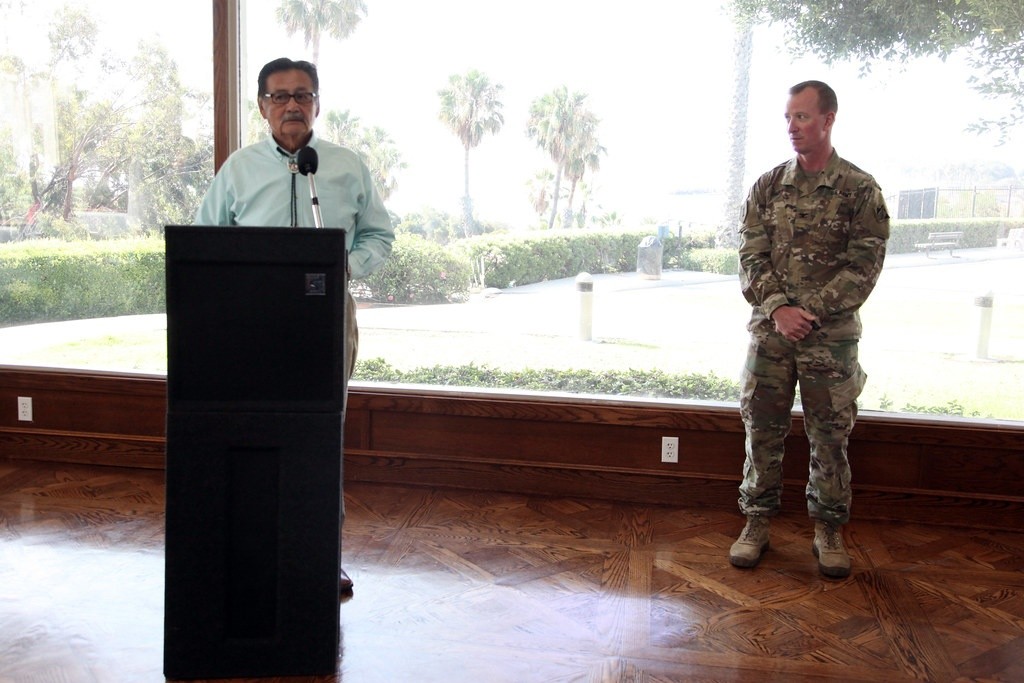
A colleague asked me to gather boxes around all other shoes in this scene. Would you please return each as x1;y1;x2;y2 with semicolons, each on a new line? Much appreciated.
340;569;354;597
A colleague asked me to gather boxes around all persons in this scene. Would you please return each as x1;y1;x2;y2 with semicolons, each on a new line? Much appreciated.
729;81;891;576
197;58;396;594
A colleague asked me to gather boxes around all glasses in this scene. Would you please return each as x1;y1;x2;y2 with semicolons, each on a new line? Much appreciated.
264;92;317;105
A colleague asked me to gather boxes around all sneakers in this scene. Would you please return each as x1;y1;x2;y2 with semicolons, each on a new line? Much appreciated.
729;515;770;567
811;522;851;578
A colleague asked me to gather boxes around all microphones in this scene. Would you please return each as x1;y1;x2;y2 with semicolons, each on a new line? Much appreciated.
297;146;324;229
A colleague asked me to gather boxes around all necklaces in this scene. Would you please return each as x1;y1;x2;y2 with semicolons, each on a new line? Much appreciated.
278;148;300;173
